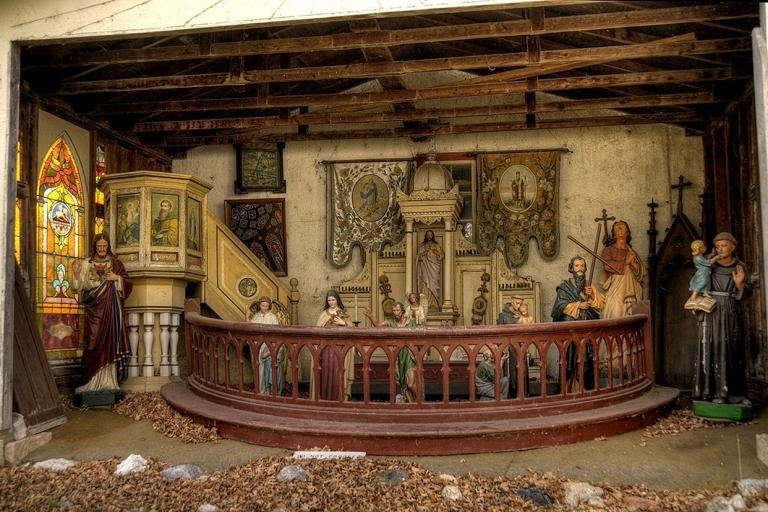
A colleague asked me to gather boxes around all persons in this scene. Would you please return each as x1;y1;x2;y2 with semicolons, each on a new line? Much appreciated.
406;293;428;325
253;297;285;394
512;171;524;201
152;198;177;247
310;291;355;402
475;222;648;402
689;232;753;404
74;234;134;385
380;302;421;402
416;230;444;308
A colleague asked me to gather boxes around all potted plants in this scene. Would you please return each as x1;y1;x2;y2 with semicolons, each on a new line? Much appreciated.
230;142;287;194
222;198;285;277
